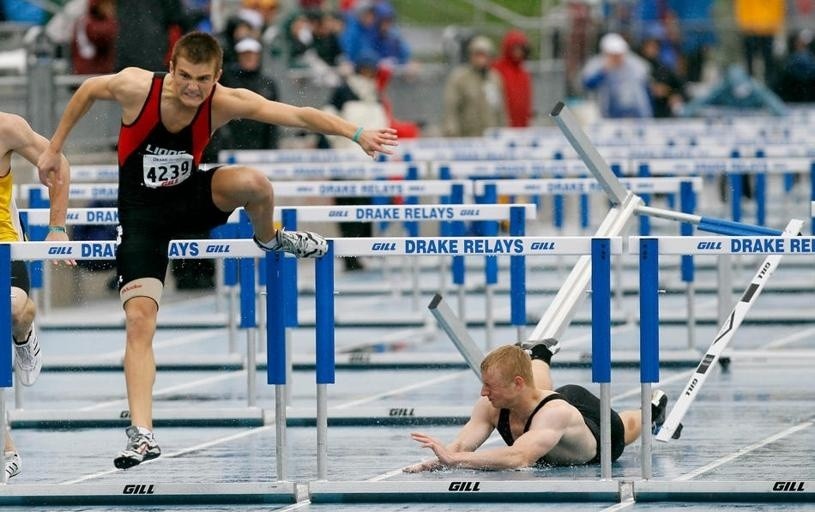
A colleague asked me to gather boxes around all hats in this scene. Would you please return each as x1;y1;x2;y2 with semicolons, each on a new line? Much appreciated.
599;31;630;56
236;38;261;52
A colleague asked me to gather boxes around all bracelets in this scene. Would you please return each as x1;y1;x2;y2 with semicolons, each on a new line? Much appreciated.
47;225;65;232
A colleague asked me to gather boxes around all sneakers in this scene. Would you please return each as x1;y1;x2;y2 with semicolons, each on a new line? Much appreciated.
514;337;560;355
114;425;161;468
4;449;24;482
252;229;329;259
652;390;668;435
13;321;42;386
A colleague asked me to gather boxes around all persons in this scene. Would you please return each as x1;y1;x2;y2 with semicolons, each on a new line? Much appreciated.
578;2;814;205
38;30;398;468
437;31;533;233
401;338;668;475
72;2;426;277
0;112;78;483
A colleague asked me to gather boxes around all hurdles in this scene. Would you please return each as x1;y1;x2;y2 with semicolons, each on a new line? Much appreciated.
6;208;268;428
429;102;807;446
470;172;710;367
25;110;813;380
282;235;625;505
225;202;540;427
627;235;815;503
1;236;301;505
